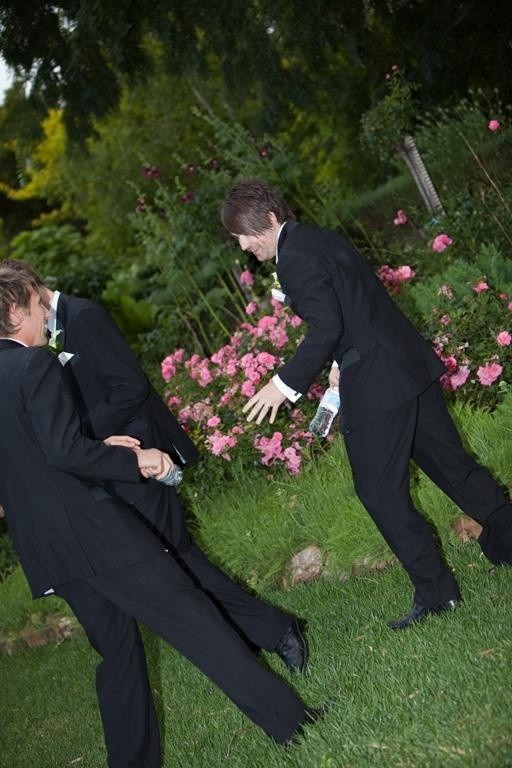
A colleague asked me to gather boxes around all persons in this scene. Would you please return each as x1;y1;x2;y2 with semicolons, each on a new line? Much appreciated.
223;179;512;629
0;269;332;766
0;254;311;683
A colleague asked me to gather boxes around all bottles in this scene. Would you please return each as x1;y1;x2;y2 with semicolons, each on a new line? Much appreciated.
309;385;341;437
158;464;183;487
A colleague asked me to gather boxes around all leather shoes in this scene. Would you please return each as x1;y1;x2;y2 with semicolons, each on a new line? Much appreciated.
388;598;459;630
272;617;310;678
